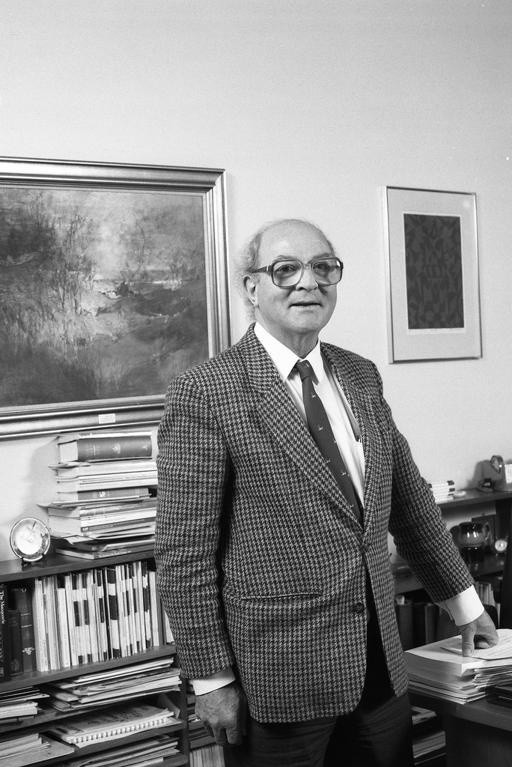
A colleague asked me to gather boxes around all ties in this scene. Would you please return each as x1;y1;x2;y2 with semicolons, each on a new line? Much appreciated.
295;360;360;522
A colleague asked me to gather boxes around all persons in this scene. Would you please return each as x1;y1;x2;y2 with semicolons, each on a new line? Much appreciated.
153;219;498;767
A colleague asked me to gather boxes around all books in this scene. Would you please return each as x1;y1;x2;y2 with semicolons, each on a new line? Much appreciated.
410;703;446;759
0;561;226;767
37;432;159;560
403;628;512;685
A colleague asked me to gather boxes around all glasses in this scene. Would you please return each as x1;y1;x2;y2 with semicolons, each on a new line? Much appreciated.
252;257;343;287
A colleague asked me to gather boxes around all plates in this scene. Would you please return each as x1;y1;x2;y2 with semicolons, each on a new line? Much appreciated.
9;517;51;563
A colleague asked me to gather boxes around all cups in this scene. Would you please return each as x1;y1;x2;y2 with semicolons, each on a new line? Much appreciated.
456;521;492;562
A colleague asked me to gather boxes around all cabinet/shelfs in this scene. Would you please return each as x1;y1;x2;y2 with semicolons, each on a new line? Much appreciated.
0;545;226;766
382;491;512;766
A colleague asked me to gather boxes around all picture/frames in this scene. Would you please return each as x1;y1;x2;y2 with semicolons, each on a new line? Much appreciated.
385;185;483;365
0;155;235;444
